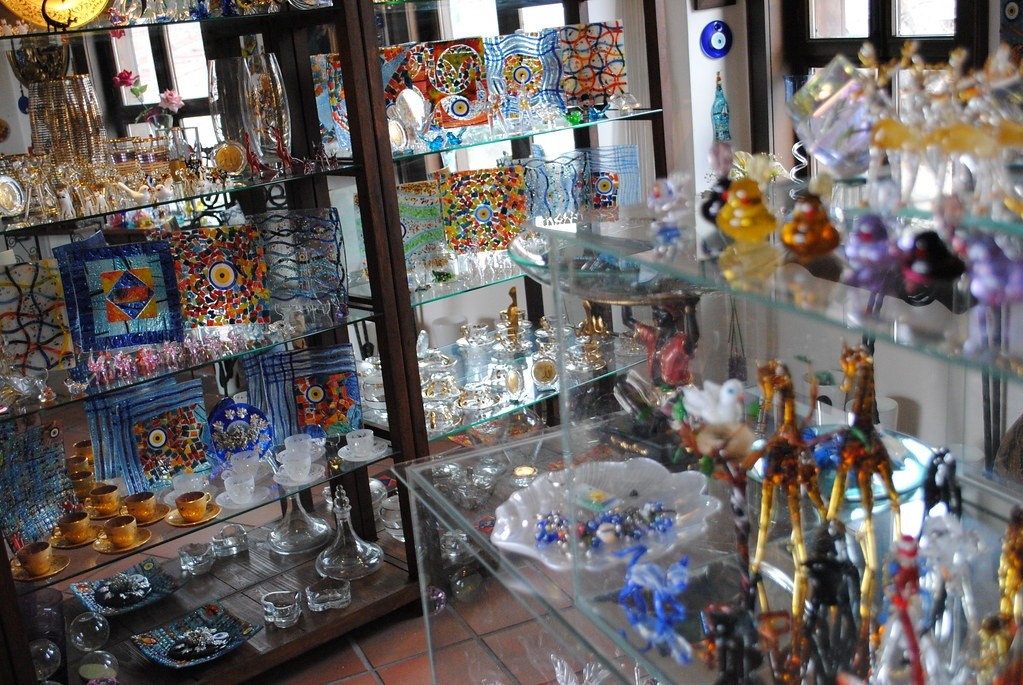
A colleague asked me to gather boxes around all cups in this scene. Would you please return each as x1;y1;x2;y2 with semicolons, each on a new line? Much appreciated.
367;309;648;422
9;439;211;574
844;396;899;431
0;53;292;201
173;429;373;504
801;371;849;410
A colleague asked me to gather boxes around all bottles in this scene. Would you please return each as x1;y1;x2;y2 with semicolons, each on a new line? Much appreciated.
268;486;385;580
712;71;732;142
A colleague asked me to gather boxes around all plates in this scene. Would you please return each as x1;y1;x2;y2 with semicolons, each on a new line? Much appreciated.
163;442;388;509
491;456;723;574
70;554;263;669
355;321;648;432
745;423;935;502
8;481;221;582
314;19;643;269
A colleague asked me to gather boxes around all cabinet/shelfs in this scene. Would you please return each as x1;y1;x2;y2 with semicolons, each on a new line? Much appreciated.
0;0;1023;683
